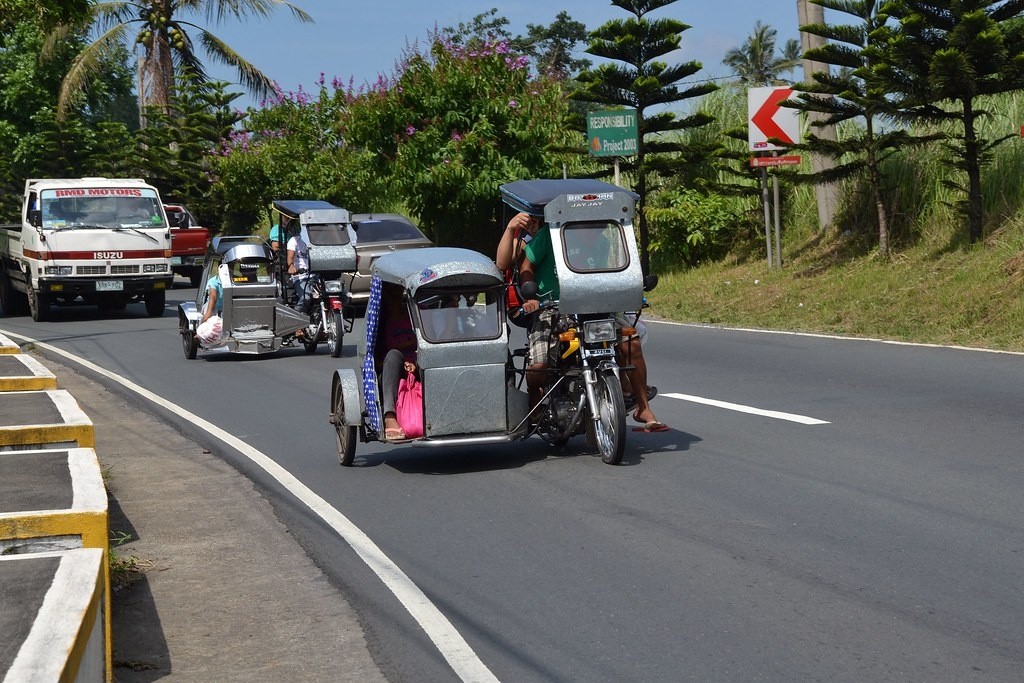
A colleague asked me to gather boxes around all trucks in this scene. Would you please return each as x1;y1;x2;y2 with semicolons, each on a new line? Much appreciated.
0;177;172;322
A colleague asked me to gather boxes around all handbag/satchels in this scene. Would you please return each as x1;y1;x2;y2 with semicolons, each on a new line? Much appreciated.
395;371;424;439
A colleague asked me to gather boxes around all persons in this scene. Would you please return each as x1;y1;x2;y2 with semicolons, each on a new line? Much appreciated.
117;198;150;219
496;212;669;433
381;287;457;440
194;208;360;342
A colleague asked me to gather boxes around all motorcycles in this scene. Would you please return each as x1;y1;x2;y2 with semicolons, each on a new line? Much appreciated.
329;179;658;466
178;200;360;360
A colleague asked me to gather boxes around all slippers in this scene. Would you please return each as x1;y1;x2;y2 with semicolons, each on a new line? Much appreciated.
632;421;671;433
384;426;406;440
530;402;547;425
295;331;305;337
646;384;657;402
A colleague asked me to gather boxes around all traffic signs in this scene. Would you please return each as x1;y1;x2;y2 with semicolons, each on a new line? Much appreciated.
748;85;802;167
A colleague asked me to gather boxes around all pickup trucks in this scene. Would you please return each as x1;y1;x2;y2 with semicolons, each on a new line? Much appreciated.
148;204;209;287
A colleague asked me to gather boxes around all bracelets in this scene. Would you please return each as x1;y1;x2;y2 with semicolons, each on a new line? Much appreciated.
289;263;293;267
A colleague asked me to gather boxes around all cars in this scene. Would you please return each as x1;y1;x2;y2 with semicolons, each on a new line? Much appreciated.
339;213;434;319
